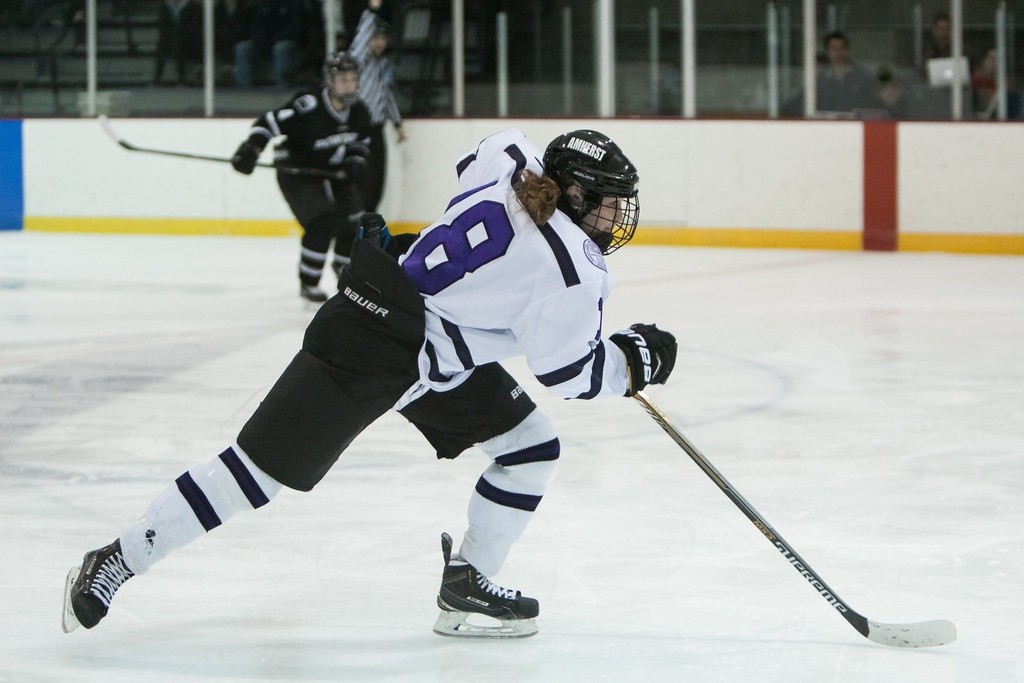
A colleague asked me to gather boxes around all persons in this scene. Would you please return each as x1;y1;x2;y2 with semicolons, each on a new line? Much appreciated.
67;124;679;640
804;19;1017;123
348;0;407;213
232;51;387;300
151;0;323;96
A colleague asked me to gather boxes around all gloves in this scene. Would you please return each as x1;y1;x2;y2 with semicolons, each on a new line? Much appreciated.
610;322;677;399
231;140;260;176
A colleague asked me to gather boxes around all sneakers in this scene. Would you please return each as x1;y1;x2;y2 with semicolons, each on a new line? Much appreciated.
62;537;135;634
299;282;326;311
434;529;540;638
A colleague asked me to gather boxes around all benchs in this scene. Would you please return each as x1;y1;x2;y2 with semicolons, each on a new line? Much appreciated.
0;0;487;117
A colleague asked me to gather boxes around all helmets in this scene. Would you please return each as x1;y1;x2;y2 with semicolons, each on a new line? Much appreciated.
543;129;638;253
323;50;363;105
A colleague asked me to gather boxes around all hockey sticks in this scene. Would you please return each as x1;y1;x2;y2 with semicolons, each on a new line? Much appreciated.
96;112;351;181
630;389;959;651
